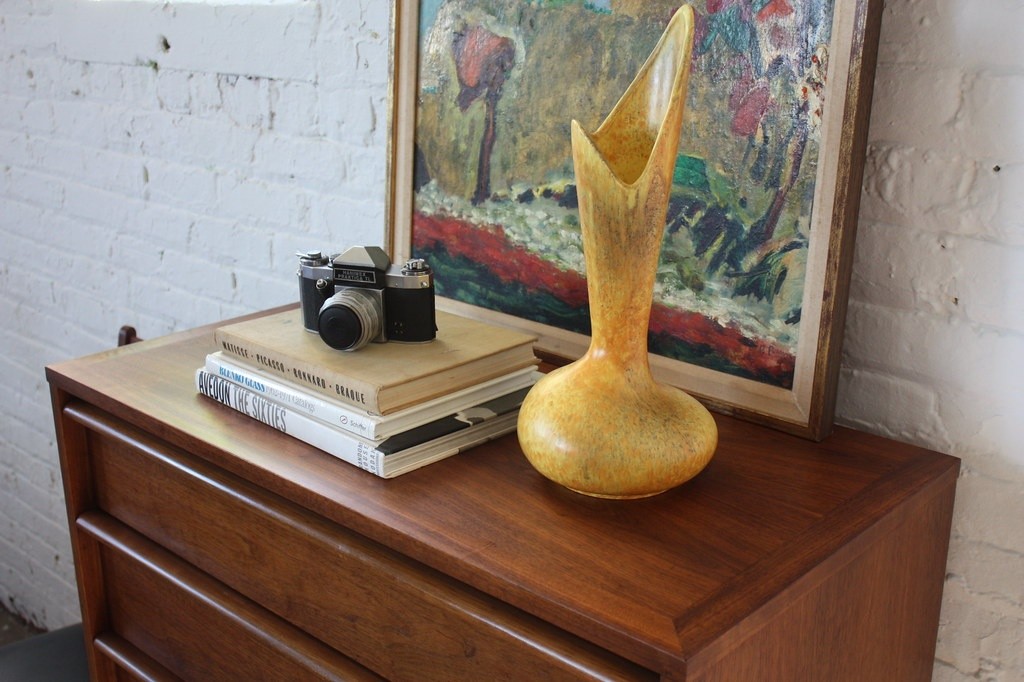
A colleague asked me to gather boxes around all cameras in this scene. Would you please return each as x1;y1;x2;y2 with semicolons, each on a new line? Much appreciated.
297;246;436;351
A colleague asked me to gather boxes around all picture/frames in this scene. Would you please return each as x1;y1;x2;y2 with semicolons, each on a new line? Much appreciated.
383;0;885;442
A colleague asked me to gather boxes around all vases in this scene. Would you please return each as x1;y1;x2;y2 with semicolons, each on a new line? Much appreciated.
517;4;717;501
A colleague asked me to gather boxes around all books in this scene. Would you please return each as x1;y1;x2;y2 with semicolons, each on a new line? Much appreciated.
197;351;548;480
215;308;543;414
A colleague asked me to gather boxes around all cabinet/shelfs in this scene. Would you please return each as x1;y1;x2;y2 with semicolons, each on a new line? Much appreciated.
46;300;963;682
